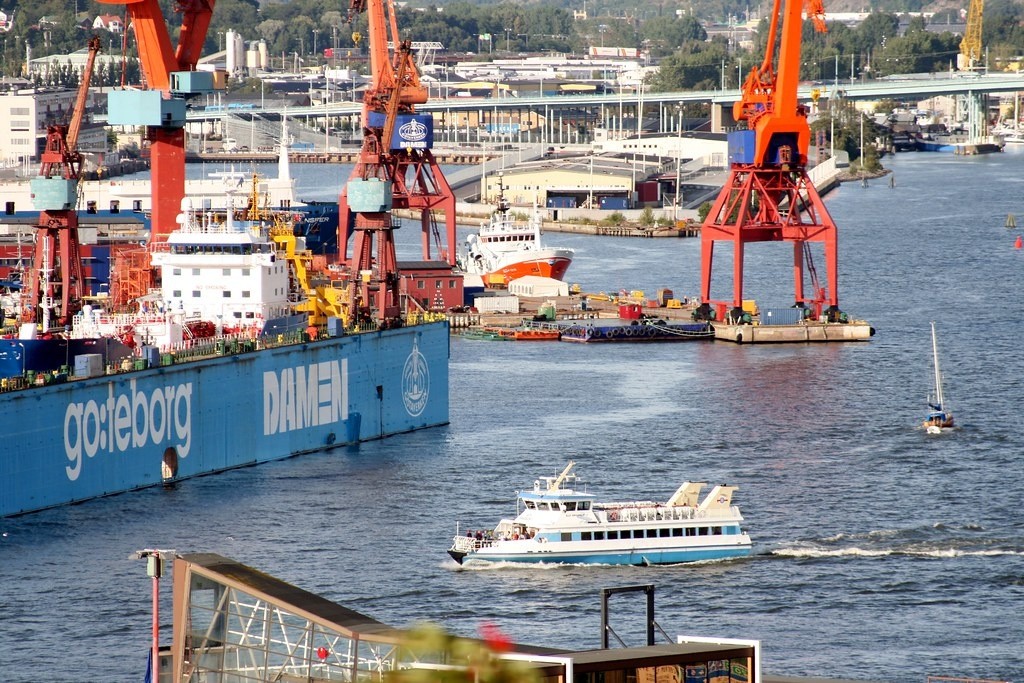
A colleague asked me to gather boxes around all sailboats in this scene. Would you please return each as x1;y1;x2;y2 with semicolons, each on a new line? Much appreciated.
924;319;955;434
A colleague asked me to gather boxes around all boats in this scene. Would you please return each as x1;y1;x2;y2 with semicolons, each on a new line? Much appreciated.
462;162;572;289
446;461;752;570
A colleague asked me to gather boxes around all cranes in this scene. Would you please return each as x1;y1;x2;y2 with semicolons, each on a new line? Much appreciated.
109;3;228;254
696;0;847;321
325;1;464;315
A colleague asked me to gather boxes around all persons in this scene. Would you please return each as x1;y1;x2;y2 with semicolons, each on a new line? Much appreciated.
465;526;531;548
